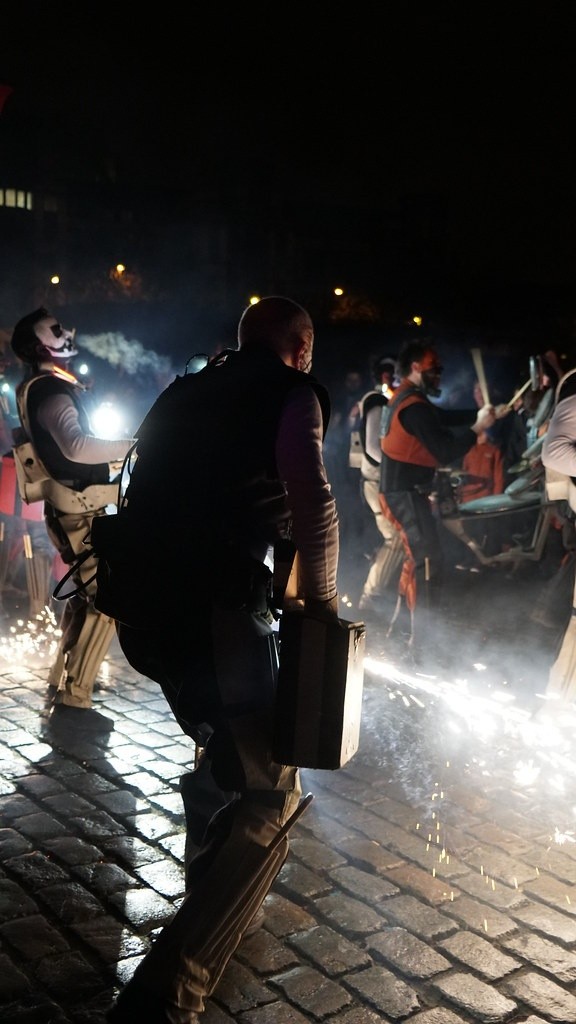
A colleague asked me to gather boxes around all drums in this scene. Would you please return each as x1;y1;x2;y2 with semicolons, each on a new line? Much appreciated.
458;355;556;515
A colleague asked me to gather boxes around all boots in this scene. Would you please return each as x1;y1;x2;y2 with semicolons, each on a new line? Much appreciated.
409;608;464;670
386;595;417;639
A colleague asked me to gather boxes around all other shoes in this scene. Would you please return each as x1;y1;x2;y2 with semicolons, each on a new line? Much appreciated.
243;905;265;941
49;704;115;732
358;595;409;613
106;987;203;1024
48;681;101;691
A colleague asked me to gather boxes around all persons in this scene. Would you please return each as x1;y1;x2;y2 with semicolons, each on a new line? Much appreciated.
328;344;576;652
89;296;342;1024
16;305;138;714
